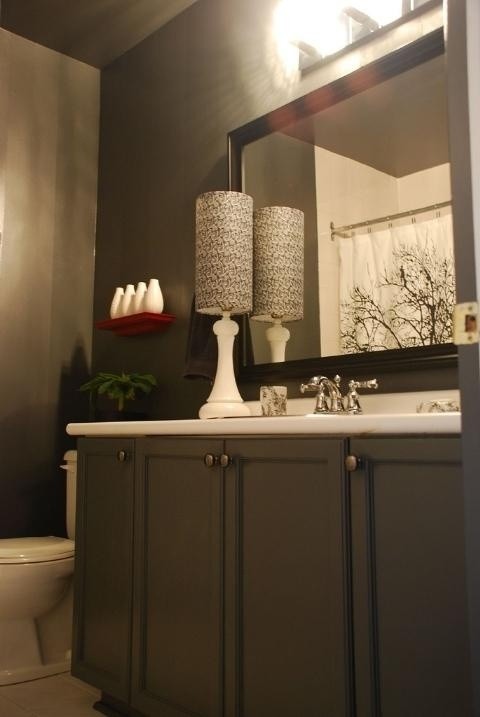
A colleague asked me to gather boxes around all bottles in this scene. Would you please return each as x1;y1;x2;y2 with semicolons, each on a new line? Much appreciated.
110;278;165;320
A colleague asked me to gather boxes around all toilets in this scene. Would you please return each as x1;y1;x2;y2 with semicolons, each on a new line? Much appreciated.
0;449;78;686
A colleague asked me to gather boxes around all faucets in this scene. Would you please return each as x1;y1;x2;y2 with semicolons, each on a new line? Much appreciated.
307;375;345;414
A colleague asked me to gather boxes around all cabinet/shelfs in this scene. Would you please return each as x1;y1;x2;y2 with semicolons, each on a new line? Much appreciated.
66;437;224;716
225;434;473;717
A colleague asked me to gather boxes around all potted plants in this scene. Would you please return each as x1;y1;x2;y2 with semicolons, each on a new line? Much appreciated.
75;368;160;422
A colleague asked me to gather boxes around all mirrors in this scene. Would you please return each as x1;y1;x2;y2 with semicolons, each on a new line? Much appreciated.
224;27;474;385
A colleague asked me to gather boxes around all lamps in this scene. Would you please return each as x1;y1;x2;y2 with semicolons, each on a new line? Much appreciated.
252;207;306;366
193;192;256;420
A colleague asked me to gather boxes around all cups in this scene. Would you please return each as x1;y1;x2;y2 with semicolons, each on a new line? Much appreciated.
259;386;288;415
